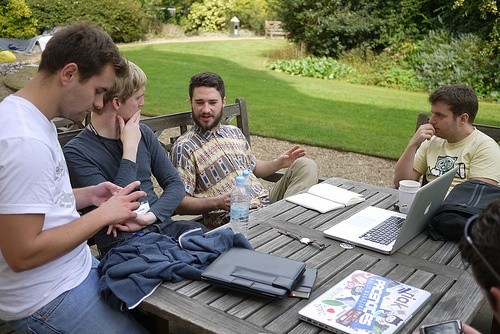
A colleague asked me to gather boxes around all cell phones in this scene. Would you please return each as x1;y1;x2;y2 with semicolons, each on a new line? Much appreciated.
419;319;465;334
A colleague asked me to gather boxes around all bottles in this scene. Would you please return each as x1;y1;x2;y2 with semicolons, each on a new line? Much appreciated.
229;176;250;239
242;171;252;201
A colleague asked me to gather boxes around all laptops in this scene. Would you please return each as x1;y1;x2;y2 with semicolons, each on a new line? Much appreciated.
321;164;459;256
296;270;431;334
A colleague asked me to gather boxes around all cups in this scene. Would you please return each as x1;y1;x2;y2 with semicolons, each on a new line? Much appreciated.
398;180;421;214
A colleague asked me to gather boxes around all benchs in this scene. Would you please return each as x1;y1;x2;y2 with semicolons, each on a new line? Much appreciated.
416;113;500;188
265;21;290;40
59;97;328;258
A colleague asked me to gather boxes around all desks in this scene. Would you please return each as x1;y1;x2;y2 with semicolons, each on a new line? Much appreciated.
139;177;500;334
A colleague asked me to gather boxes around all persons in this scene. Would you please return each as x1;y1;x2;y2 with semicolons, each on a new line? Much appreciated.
459;199;500;334
169;72;318;230
62;58;208;260
0;23;155;334
393;84;500;200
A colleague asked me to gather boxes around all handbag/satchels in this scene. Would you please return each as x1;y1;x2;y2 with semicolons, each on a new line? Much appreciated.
424;179;500;243
